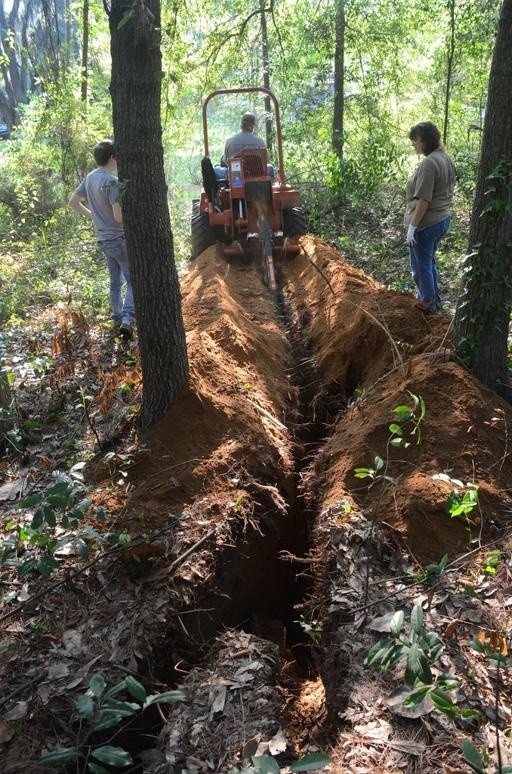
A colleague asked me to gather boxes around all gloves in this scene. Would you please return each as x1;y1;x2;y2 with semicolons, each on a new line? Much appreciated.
406;224;417;247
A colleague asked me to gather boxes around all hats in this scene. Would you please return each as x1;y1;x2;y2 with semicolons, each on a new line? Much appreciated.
241;113;256;125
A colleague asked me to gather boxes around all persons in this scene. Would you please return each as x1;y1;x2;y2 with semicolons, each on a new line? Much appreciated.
223;114;270;160
68;138;140;339
401;121;457;312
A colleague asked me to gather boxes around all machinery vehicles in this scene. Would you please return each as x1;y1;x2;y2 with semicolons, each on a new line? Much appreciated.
188;85;310;300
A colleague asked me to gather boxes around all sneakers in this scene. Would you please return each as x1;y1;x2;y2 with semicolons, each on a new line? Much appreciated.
120;325;135;342
